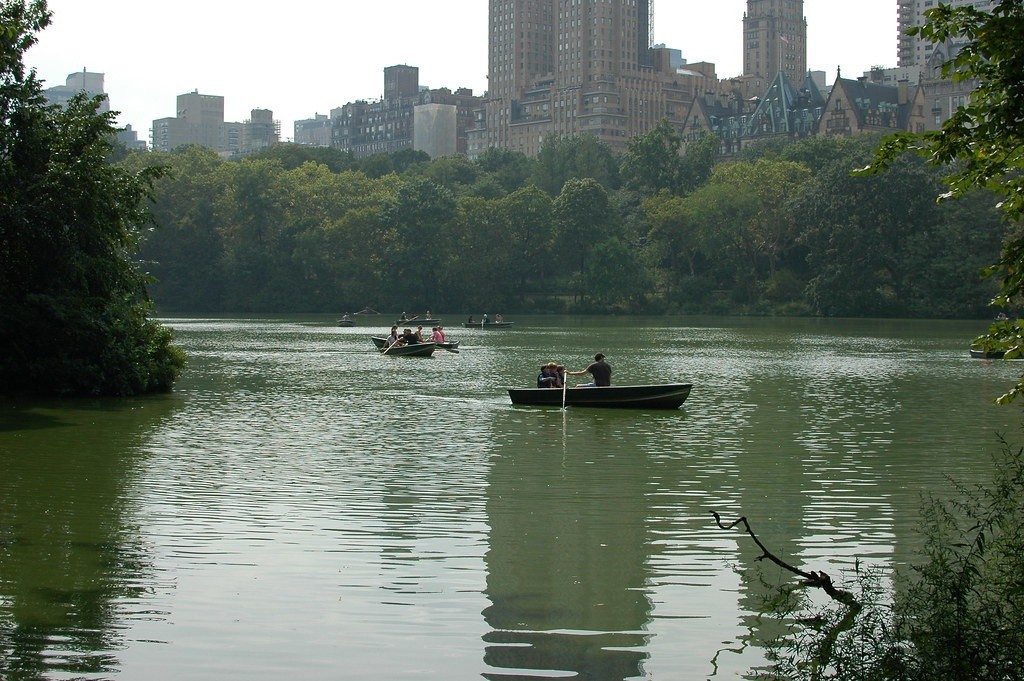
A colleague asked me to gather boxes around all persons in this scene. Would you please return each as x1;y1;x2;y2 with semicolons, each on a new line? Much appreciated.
495;313;504;323
415;325;424;342
401;312;406;320
537;362;567;388
437;326;446;341
343;312;349;320
563;353;611;386
469;316;472;323
383;325;418;348
481;314;490;323
431;327;443;343
425;311;432;319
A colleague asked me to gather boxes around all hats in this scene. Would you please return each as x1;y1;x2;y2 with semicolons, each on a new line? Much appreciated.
594;353;605;361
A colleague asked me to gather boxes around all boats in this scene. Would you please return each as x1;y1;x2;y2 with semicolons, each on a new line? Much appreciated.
460;321;515;328
354;307;381;316
969;350;1006;359
506;382;693;409
393;318;442;326
370;335;459;357
337;319;355;327
